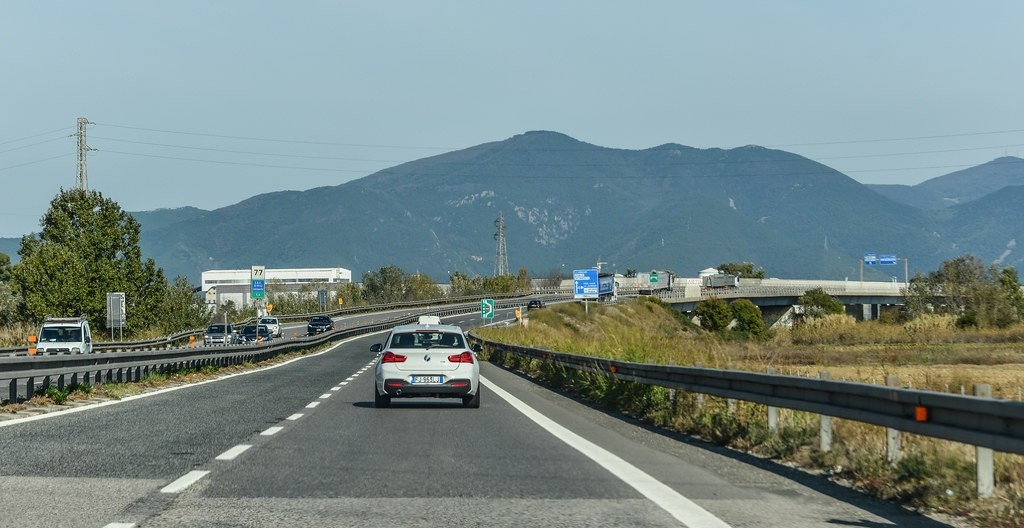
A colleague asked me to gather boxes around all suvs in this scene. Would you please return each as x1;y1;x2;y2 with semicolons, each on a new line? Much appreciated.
38;318;92;355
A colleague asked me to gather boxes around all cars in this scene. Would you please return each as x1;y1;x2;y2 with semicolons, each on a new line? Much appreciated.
370;315;483;408
205;314;334;345
528;301;546;310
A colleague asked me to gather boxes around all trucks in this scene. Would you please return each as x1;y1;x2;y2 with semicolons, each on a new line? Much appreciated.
703;275;740;290
639;272;674;294
583;274;618;301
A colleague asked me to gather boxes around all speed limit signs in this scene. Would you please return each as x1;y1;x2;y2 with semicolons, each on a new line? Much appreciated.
252;266;264;279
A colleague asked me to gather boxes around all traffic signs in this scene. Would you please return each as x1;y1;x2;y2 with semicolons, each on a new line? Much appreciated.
573;270;598;298
